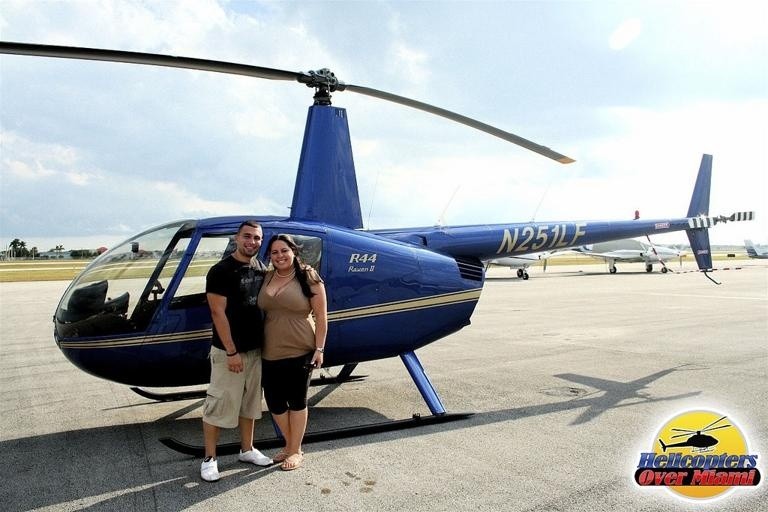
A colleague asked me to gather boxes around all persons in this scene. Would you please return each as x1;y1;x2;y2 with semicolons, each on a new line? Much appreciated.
198;220;313;483
255;231;328;473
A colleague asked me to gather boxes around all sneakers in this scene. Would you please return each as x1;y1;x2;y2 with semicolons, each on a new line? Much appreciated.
239;447;273;466
200;456;220;482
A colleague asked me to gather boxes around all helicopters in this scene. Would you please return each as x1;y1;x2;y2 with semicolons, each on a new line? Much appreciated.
659;414;732;457
0;40;753;454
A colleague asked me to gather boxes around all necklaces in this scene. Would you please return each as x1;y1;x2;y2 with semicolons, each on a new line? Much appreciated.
275;267;296;278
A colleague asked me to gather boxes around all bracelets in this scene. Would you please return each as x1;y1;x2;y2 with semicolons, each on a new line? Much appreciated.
315;347;324;353
226;350;237;358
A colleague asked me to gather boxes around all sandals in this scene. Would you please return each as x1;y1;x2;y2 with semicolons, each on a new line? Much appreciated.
273;448;304;471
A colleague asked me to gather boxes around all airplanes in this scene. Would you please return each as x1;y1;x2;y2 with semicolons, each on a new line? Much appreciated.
744;239;768;258
582;238;687;273
481;243;594;280
